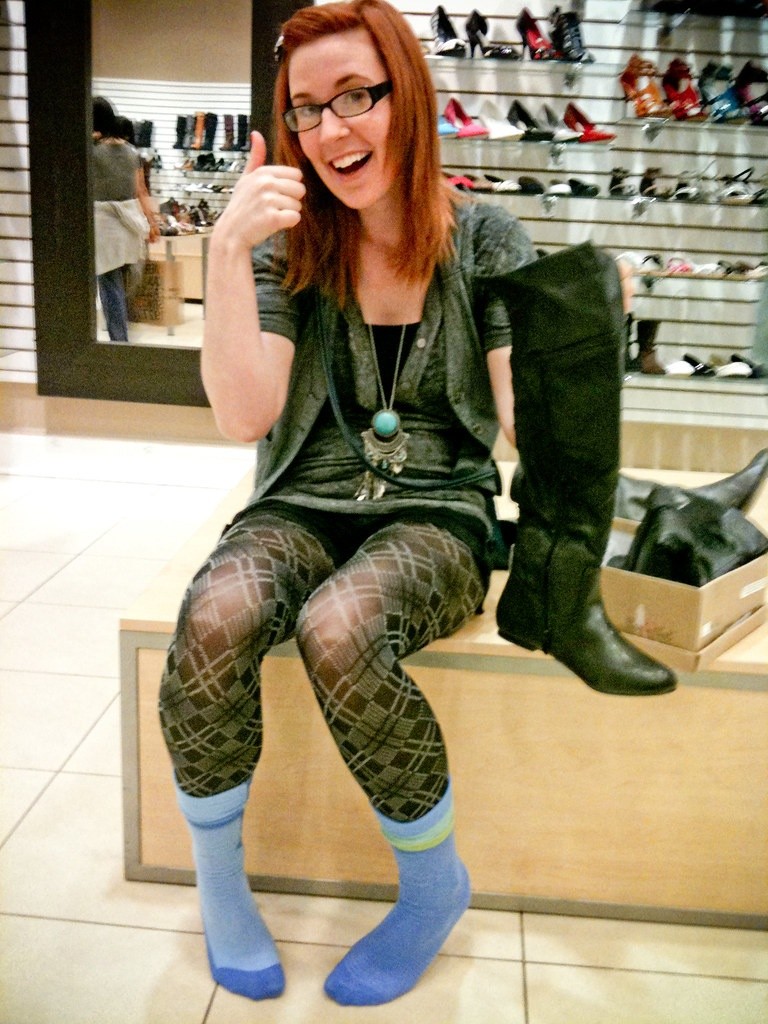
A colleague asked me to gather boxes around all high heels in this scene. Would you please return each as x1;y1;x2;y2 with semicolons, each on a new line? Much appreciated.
431;5;768;379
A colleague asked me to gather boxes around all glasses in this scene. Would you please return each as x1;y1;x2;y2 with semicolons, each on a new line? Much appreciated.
282;80;392;133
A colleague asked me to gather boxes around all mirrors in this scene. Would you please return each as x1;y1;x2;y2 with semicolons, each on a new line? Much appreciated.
91;0;252;348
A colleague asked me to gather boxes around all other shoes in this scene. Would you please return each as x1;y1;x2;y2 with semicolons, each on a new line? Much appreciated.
155;153;249;236
605;483;768;588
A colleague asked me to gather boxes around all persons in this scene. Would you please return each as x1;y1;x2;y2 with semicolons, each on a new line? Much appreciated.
90;98;160;341
157;0;633;1005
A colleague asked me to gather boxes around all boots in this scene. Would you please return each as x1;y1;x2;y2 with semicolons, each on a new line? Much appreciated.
470;240;676;695
509;446;768;524
133;113;252;150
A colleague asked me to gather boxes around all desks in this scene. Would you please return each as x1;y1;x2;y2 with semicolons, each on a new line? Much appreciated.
147;230;211;300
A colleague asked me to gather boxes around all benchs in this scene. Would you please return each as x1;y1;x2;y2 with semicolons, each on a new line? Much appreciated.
117;466;768;931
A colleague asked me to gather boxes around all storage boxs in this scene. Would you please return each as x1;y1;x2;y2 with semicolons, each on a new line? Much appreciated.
598;515;768;677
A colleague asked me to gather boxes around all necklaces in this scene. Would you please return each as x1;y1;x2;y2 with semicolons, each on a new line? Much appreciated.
357;265;417;499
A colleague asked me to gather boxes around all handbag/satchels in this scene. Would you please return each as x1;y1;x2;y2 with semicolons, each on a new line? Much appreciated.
123;237;185;327
